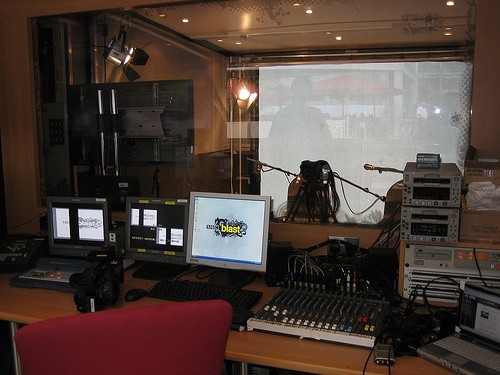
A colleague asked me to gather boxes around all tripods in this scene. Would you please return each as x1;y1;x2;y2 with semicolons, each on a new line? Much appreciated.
284;182;338;224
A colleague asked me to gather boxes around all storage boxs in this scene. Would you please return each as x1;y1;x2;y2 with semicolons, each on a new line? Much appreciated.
460;194;500;243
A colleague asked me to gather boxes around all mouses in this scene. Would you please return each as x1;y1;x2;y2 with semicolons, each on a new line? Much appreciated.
124;288;147;301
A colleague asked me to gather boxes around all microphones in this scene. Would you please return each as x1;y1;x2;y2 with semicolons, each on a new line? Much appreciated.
380;196;386;202
364;164;375;170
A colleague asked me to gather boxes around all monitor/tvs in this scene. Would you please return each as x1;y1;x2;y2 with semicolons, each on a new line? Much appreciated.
65;80;196;167
125;197;190;281
46;196;110;257
186;192;271;288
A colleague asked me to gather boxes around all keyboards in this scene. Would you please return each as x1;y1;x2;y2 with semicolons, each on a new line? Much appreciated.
147;279;263;311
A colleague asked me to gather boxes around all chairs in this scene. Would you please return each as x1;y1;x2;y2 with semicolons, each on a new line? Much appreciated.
14;299;234;375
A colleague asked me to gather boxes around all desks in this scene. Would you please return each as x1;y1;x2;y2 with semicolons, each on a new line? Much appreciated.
0;262;456;375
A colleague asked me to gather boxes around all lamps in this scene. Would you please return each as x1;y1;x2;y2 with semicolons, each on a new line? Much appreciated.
233;79;250;100
101;24;150;83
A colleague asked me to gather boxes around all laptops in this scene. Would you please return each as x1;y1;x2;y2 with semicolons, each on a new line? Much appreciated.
416;280;500;375
